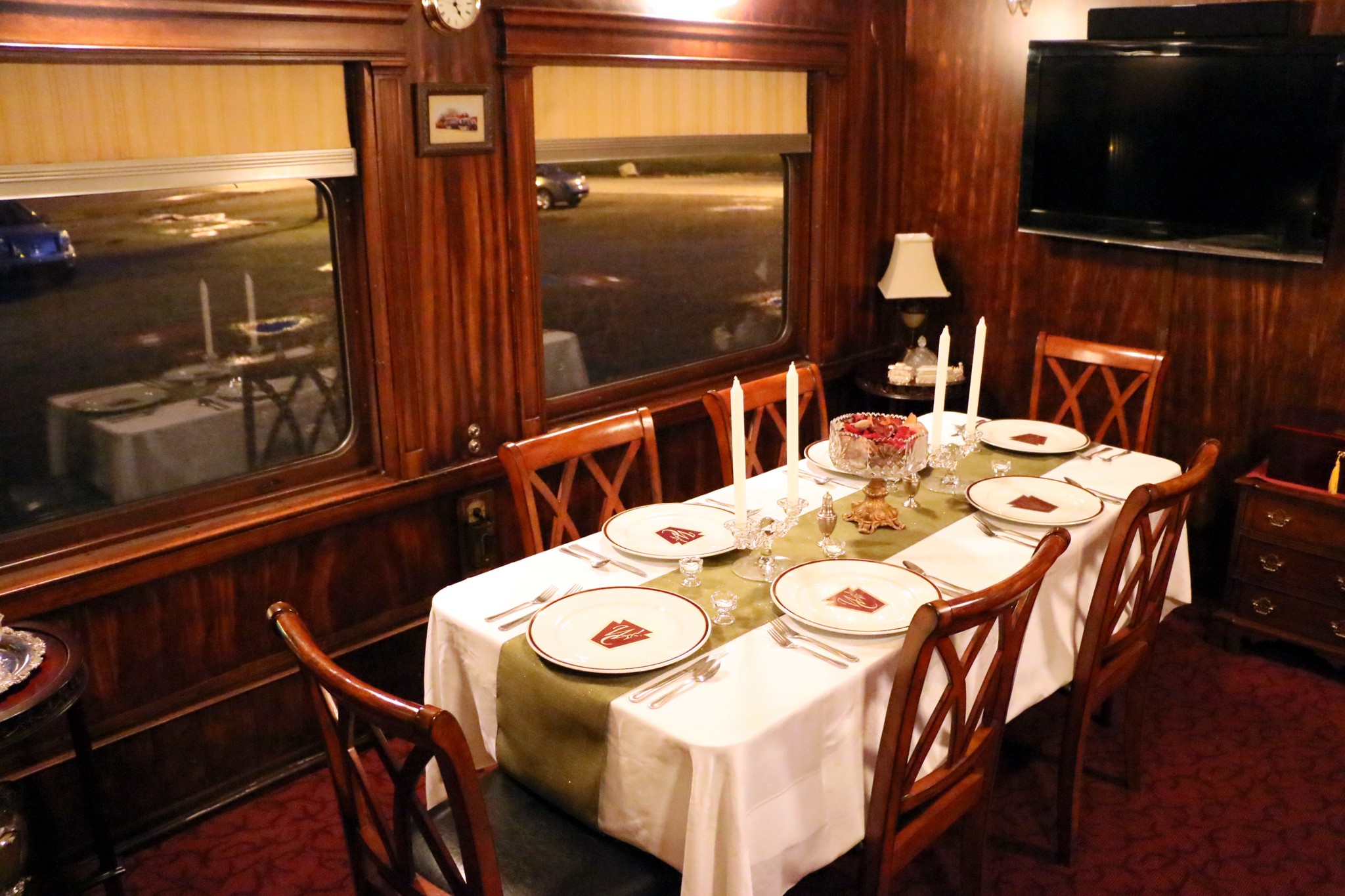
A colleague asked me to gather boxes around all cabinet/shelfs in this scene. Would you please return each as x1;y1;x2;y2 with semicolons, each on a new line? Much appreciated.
1213;456;1345;671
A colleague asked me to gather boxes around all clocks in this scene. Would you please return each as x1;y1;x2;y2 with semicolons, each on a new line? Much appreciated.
420;0;483;36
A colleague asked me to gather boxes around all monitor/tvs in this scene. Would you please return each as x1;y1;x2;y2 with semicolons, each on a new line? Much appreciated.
1016;33;1345;264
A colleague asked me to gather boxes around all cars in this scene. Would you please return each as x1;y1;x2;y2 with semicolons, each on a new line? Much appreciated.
536;163;589;211
0;199;77;277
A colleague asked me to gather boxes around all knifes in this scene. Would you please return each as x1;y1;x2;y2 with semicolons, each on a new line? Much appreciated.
568;544;647;577
629;655;710;703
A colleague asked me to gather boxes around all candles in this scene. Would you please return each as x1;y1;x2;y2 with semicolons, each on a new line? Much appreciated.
786;359;799;507
964;314;988;436
729;374;748;531
243;271;262;357
197;275;217;361
929;323;952;454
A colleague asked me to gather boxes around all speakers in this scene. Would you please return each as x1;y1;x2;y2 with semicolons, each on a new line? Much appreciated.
1088;1;1317;41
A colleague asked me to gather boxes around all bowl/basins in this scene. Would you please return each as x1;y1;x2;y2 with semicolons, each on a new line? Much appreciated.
904;334;938;377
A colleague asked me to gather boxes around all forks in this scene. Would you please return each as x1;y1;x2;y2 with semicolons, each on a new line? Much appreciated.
706;499;763;515
1098;450;1130;462
485;586;559;622
976;522;1037;549
1075;448;1113;460
767;627;849;668
973;512;1041;542
769;612;859;662
498;584;583;631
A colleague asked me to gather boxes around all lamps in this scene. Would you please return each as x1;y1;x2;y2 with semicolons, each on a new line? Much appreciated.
876;231;951;363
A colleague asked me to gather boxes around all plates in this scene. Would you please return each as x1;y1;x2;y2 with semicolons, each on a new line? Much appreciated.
165;363;233;382
965;475;1105;526
976;418;1090;453
525;586;711;673
601;503;736;559
74;385;169;412
803;437;927;478
770;558;943;635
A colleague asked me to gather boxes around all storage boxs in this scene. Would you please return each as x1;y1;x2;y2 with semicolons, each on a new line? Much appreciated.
1266;408;1345;495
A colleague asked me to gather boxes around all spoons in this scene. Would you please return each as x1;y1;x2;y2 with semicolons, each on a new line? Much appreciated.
649;659;721;709
903;560;974;595
783;471;833;485
1065;477;1127;501
560;547;610;568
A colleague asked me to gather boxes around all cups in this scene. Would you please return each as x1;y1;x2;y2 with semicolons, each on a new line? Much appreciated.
679;556;703;586
823;539;846;557
711;590;738;626
992;459;1011;477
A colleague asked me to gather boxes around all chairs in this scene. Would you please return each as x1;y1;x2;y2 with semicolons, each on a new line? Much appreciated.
800;521;1071;896
1010;436;1224;870
701;358;833;489
240;351;353;474
501;404;670;558
1025;328;1172;459
263;599;680;896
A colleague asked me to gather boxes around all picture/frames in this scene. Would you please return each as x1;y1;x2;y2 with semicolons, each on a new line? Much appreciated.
408;80;495;157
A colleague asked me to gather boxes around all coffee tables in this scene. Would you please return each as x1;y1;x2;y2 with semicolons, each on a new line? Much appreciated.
1;622;128;896
855;370;971;419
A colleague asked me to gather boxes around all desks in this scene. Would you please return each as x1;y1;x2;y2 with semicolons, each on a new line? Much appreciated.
47;329;588;510
424;409;1192;896
